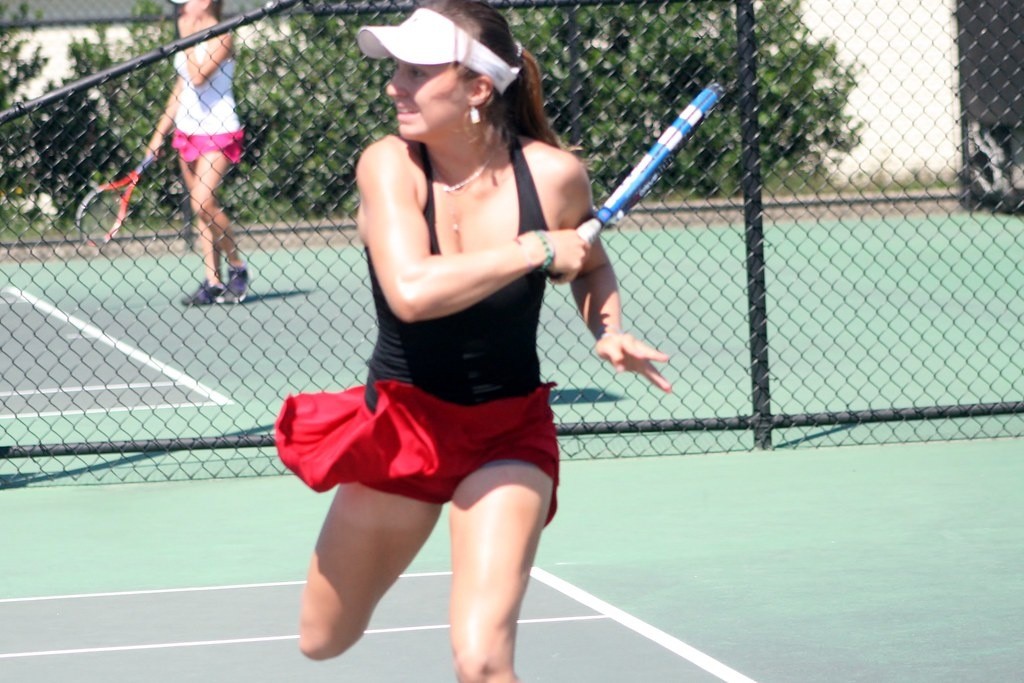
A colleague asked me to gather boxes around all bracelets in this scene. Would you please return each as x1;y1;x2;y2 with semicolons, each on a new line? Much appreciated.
596;322;623;341
515;229;555;272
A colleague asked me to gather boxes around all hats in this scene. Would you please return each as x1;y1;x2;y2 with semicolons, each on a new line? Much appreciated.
354;6;521;98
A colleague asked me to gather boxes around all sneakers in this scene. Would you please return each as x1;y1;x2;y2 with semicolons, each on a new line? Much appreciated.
216;263;250;302
181;279;228;307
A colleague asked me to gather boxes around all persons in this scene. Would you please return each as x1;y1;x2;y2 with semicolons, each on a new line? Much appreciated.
145;0;248;306
274;0;671;683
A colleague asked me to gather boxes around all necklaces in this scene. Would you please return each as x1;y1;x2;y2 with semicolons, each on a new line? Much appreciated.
428;140;498;231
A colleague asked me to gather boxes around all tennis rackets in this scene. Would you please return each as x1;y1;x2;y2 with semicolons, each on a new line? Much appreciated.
74;152;157;251
536;78;730;279
